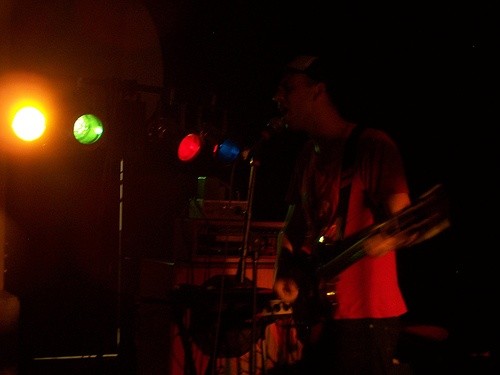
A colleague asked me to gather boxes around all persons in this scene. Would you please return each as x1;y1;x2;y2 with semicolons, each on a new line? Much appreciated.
271;52;413;375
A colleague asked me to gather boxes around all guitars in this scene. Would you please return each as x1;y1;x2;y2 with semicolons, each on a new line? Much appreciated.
274;181;450;354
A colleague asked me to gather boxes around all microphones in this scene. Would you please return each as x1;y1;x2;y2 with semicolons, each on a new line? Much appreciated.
246;117;283;161
